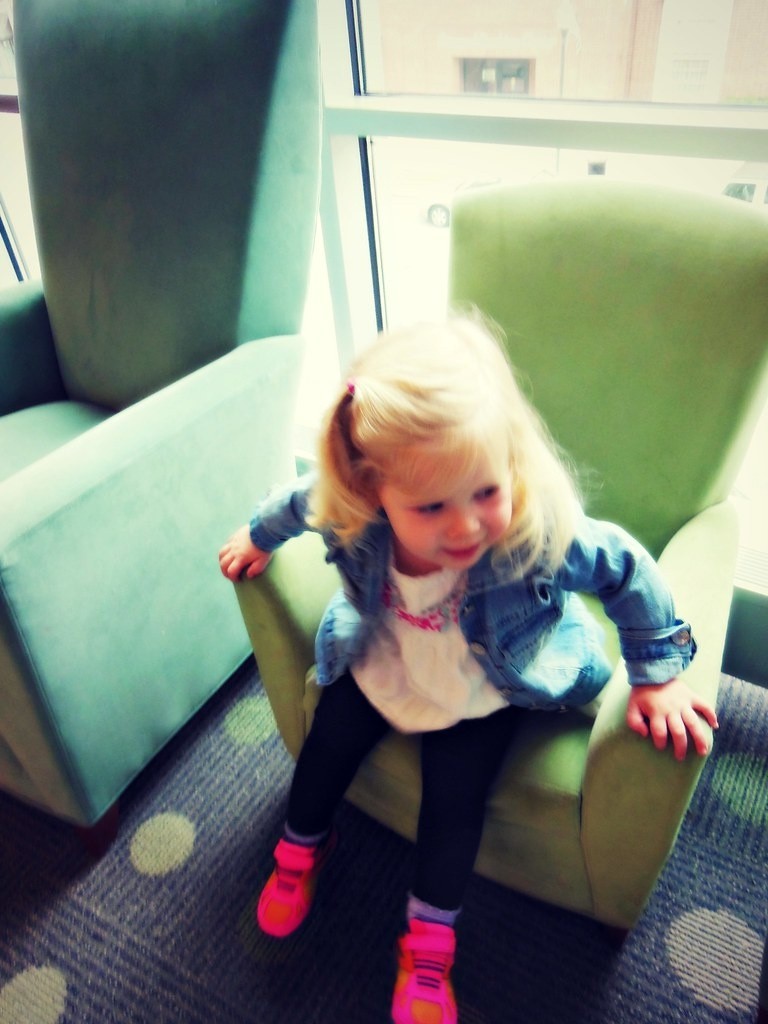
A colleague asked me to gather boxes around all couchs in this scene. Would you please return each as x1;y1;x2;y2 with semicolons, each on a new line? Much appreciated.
0;0;322;829
234;176;767;927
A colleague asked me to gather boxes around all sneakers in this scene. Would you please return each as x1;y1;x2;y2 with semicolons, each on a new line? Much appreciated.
255;835;318;938
390;917;461;1024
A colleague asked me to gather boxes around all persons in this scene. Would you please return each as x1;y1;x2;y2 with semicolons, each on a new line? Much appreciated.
220;304;719;1024
